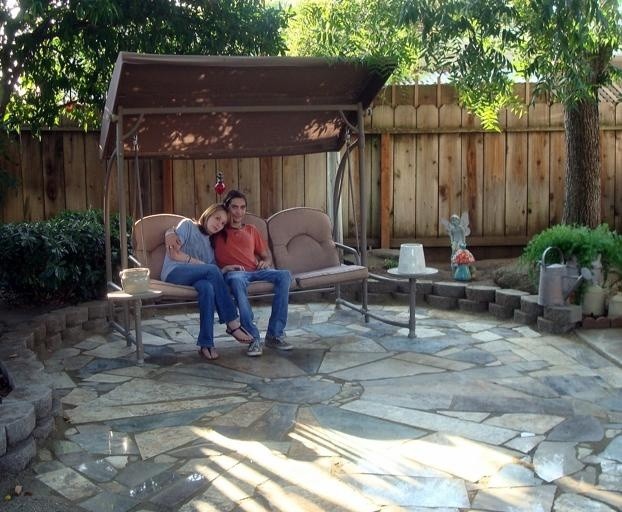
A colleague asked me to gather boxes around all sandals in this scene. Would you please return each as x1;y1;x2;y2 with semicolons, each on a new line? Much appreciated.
200;346;218;360
226;325;254;343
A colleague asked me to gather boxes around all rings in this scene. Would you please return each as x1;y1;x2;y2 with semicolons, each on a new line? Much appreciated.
166;245;169;248
169;246;173;249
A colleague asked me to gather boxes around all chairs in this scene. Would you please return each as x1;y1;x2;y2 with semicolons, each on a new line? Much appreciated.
131;207;369;301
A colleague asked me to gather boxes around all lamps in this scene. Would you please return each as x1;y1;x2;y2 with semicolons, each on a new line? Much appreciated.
214;171;226;194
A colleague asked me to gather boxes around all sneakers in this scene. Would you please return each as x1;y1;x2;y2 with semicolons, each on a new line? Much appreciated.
247;337;263;356
265;335;293;350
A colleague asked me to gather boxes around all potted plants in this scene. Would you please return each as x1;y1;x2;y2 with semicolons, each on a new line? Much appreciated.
513;222;622;291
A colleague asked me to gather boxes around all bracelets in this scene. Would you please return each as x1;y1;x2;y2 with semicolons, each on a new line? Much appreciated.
185;253;192;263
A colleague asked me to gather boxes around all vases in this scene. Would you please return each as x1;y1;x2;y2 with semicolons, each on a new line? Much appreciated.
398;244;426;273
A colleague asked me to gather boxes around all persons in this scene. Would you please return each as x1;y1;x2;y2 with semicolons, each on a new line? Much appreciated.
440;211;471;274
159;203;253;360
165;191;293;358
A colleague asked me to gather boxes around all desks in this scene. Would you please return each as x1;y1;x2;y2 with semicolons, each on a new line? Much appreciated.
387;267;439;339
106;290;164;363
